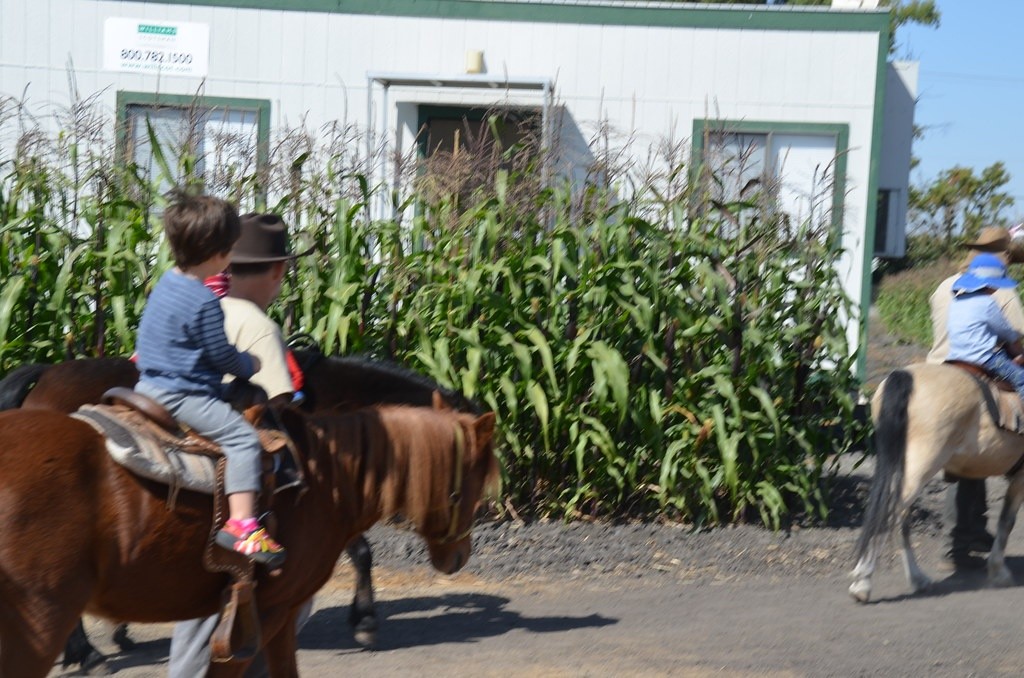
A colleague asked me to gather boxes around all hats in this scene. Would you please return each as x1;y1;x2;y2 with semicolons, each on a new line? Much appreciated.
959;227;1017;268
953;253;1018;294
230;213;318;263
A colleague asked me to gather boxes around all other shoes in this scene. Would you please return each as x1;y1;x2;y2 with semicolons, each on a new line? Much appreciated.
216;518;285;566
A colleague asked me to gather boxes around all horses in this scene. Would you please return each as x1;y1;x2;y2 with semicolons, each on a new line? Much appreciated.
848;338;1024;605
0;390;503;678
0;350;483;677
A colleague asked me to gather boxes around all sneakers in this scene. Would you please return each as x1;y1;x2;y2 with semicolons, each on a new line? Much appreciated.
972;531;995;552
937;554;985;573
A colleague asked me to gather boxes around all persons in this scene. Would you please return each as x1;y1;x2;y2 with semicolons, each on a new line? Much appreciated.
135;195;291;564
169;212;317;678
925;223;1024;565
